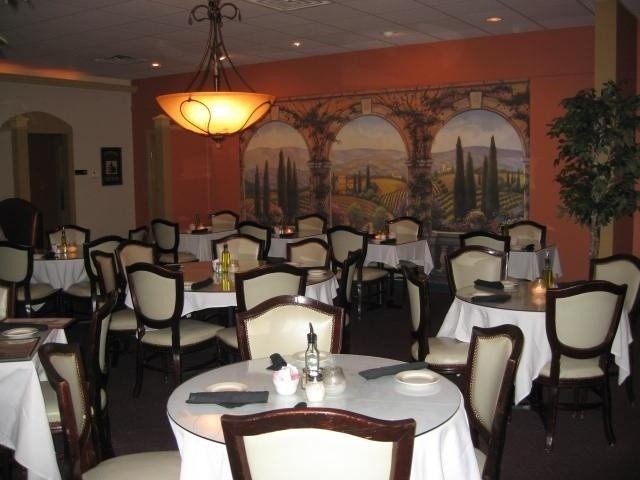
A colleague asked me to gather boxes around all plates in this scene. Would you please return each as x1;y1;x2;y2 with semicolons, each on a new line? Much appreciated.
308;275;327;279
1;327;39;339
4;337;36;344
308;270;327;274
472;293;495;297
204;382;247;393
499;281;519;288
394;369;441;385
293;350;331;362
396;384;441;396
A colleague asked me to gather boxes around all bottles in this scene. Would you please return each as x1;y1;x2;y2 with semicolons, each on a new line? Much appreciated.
61;229;67;255
306;333;322;378
221;273;231;291
383;220;389;240
542;251;553;289
281;217;287;234
222;243;229;273
194;213;200;225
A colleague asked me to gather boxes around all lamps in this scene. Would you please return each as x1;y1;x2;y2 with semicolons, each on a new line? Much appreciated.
156;0;276;149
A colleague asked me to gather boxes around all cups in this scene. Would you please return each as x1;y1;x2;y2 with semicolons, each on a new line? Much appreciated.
188;223;196;232
229;274;235;283
323;365;345;395
389;232;397;240
229;264;239;274
212;257;219;271
306;373;325;402
213;272;220;284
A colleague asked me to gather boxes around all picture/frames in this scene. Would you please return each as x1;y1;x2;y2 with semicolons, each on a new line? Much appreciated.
101;148;122;185
